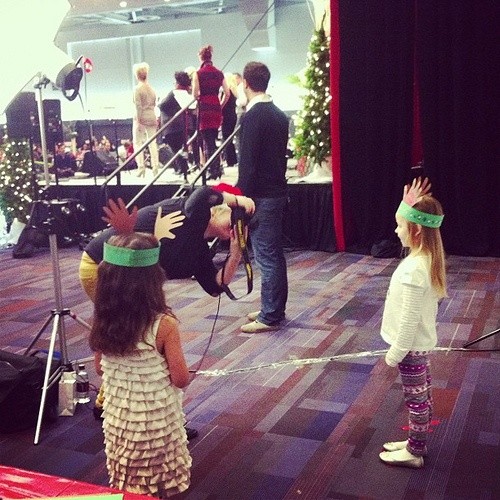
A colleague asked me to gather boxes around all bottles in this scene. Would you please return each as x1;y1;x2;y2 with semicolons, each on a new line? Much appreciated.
76;364;91;404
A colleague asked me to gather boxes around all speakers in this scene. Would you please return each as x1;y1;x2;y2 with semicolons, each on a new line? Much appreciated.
79;149;119;176
159;147;176;168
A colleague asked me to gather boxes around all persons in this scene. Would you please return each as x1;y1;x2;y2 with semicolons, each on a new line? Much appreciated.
79;183;256;440
379;176;448;466
234;62;289;334
89;199;192;497
34;44;248;181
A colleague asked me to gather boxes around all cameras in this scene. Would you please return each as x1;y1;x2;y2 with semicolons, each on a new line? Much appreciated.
231;207;259;234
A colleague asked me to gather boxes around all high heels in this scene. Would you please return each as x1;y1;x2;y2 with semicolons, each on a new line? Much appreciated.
135;168;146;177
152;168;159;177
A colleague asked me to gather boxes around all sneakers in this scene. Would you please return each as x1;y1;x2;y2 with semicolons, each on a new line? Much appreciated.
241;319;280;333
247;311;264;320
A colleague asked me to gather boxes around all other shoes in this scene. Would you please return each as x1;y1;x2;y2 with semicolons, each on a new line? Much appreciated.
93;405;105;420
379;448;424;467
184;427;198;440
383;440;428;456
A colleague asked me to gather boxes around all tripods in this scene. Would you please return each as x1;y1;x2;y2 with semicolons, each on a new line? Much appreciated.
24;232;92;446
